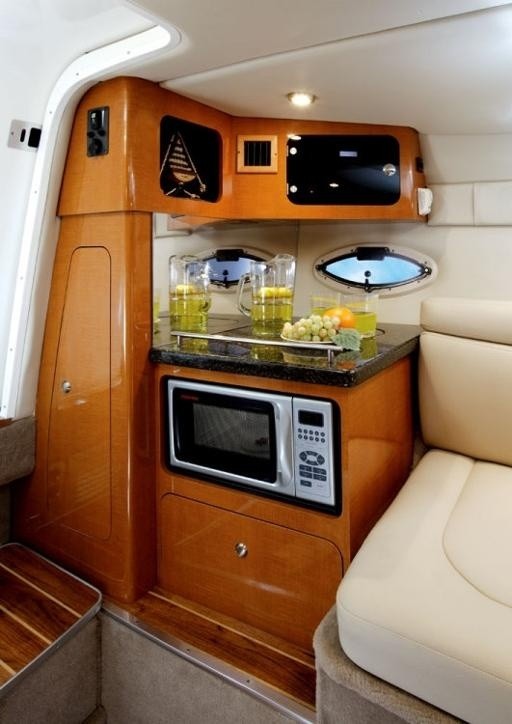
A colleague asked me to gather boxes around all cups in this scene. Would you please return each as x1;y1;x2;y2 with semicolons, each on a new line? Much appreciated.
310;290;379;339
234;260;299;326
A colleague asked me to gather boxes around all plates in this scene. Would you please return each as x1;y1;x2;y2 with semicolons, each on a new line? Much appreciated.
278;333;338;346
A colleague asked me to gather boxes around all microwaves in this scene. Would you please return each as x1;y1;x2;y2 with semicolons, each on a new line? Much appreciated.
166;377;339;511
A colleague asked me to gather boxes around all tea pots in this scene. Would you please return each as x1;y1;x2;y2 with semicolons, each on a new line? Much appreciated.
168;258;213;333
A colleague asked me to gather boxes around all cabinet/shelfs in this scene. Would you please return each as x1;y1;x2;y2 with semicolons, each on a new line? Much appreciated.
10;211;155;604
150;344;415;657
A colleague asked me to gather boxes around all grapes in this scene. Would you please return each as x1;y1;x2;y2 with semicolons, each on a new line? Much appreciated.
282;315;340;342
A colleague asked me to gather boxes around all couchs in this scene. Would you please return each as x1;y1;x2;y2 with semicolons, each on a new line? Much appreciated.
310;296;512;724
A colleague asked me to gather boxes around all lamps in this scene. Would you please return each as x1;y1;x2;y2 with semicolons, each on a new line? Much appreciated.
286;91;317;108
